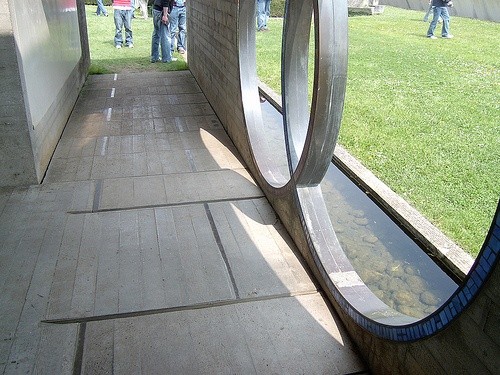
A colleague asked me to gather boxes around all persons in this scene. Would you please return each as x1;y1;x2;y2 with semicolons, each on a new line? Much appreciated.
169;0;187;54
150;0;178;63
112;0;134;49
130;0;135;19
95;0;109;17
256;0;272;32
425;0;454;39
60;0;81;50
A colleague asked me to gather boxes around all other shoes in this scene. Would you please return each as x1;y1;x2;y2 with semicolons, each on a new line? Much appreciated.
105;13;108;17
129;45;134;48
116;45;121;48
150;56;162;62
430;36;438;39
171;58;178;62
443;35;454;39
97;15;99;16
179;50;185;54
260;26;271;31
